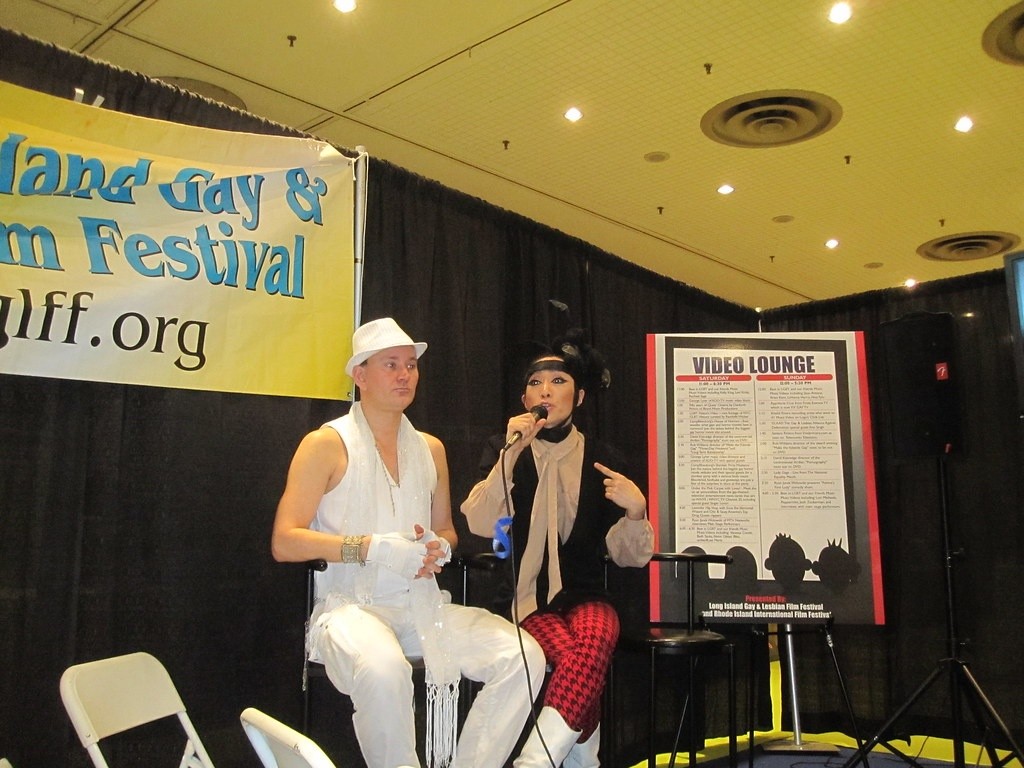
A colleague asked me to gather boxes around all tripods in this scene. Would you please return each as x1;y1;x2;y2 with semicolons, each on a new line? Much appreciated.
842;458;1024;768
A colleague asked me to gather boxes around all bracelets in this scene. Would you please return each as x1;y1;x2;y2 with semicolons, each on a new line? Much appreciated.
341;534;365;564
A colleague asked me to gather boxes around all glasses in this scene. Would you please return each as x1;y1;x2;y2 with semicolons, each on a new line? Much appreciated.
561;721;600;768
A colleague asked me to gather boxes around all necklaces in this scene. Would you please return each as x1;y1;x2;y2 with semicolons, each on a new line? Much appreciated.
375;438;401;518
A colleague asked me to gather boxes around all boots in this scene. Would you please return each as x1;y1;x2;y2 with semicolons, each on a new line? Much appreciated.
511;704;582;768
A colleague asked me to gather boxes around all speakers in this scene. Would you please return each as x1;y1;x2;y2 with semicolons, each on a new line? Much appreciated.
879;309;967;458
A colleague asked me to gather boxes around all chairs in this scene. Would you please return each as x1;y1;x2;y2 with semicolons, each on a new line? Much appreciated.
59;652;216;768
240;707;335;768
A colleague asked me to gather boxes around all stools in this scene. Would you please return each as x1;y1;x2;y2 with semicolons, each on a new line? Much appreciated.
302;552;738;768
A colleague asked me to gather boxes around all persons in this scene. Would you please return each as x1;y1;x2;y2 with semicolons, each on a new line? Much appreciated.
271;317;546;768
460;334;655;768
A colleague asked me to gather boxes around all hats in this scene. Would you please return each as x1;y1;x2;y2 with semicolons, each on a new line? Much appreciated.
344;318;427;377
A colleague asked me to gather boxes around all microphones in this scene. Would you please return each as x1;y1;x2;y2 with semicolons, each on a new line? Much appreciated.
503;405;548;452
